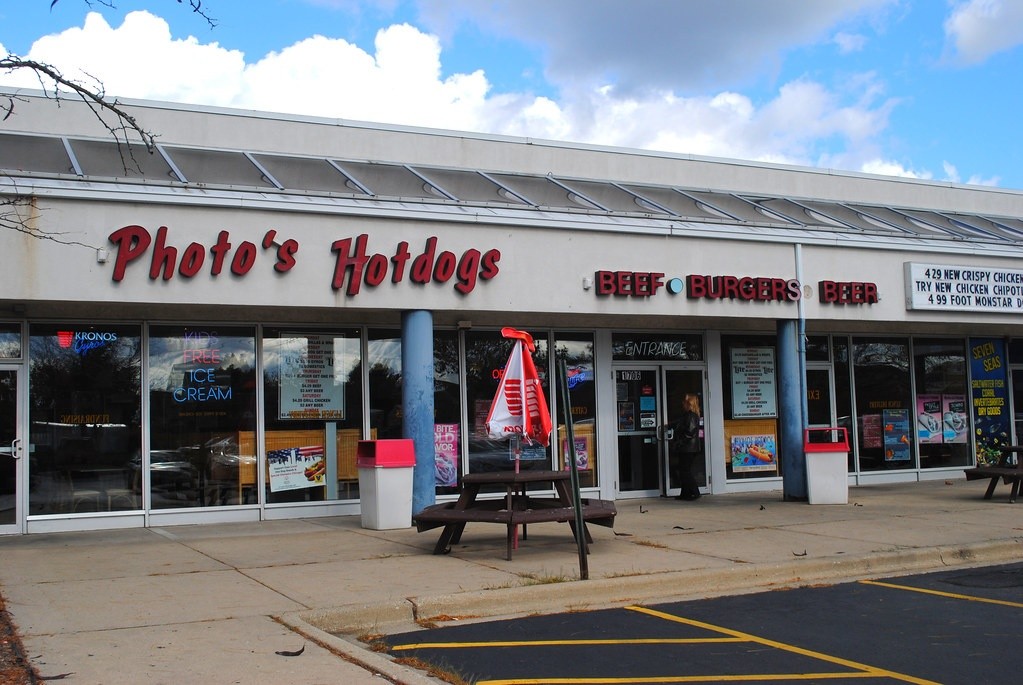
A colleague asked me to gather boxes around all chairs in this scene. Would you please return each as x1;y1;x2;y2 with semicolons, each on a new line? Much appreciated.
104;463;141;511
58;464;100;513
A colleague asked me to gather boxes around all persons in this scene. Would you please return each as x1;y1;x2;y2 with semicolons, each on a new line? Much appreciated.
665;394;703;501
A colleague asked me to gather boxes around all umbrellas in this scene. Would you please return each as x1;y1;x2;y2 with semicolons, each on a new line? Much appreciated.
483;327;553;552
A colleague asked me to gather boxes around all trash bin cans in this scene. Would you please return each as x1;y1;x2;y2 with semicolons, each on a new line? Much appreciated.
804;425;849;506
355;438;417;530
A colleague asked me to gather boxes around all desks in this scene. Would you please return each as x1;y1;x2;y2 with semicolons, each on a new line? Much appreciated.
433;469;594;554
71;464;130;511
984;445;1023;504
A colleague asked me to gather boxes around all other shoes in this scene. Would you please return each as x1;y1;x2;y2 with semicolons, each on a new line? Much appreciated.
689;493;702;501
675;496;689;500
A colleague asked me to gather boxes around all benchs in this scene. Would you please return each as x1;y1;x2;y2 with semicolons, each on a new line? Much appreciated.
511;505;617;524
966;464;1019;481
414;508;512;525
963;467;1023;478
